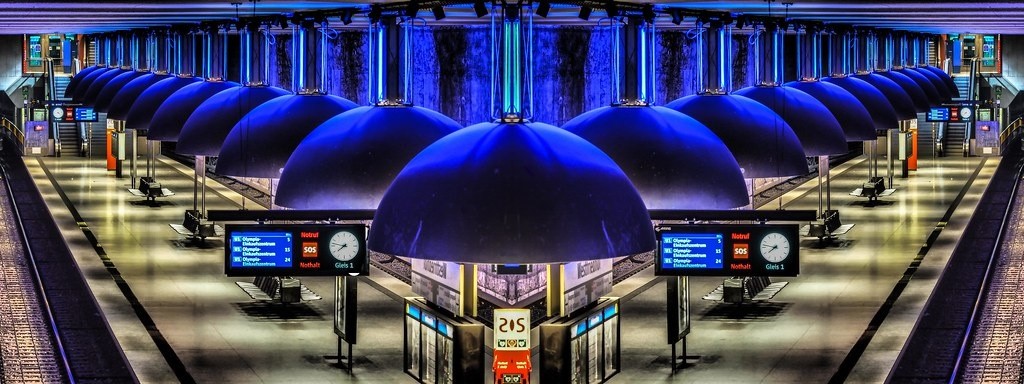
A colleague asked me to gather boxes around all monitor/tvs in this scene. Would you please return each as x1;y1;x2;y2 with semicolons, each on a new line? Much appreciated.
654;225;800;277
225;223;370;276
926;106;974;122
50;105;99;122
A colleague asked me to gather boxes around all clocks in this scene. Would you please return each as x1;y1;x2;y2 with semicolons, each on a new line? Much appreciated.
760;232;790;262
329;231;359;261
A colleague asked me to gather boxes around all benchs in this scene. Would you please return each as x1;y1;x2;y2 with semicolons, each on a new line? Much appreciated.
235;276;322;319
127;176;175;204
849;177;896;203
169;210;225;245
799;210;856;241
702;276;789;308
